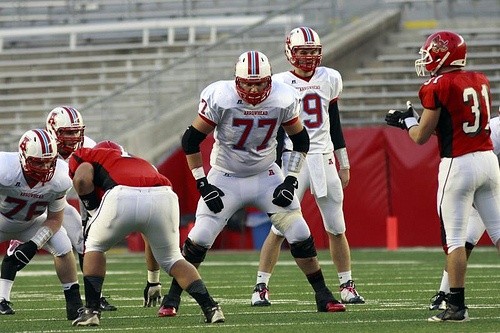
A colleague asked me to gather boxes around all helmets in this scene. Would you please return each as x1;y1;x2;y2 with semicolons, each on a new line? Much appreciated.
18;129;57;172
46;106;84;144
93;140;124;151
424;31;467;71
285;27;322;64
235;51;271;85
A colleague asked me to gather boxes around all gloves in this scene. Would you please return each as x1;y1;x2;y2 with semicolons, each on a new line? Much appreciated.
142;280;162;308
196;176;225;214
11;240;38;271
272;175;299;207
385;101;421;132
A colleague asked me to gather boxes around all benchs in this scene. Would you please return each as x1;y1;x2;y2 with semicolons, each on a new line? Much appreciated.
1;0;305;152
337;29;500;125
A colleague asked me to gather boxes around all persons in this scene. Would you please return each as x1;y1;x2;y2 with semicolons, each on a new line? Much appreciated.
65;136;226;327
0;105;118;316
159;51;347;317
385;32;500;323
429;117;500;311
250;28;365;307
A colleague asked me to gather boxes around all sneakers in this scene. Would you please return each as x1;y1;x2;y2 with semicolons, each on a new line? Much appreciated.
427;306;471;322
0;296;15;315
340;279;365;304
315;292;346;312
429;291;450;310
71;306;100;326
100;295;117;311
204;305;226;323
157;295;181;317
251;283;271;306
